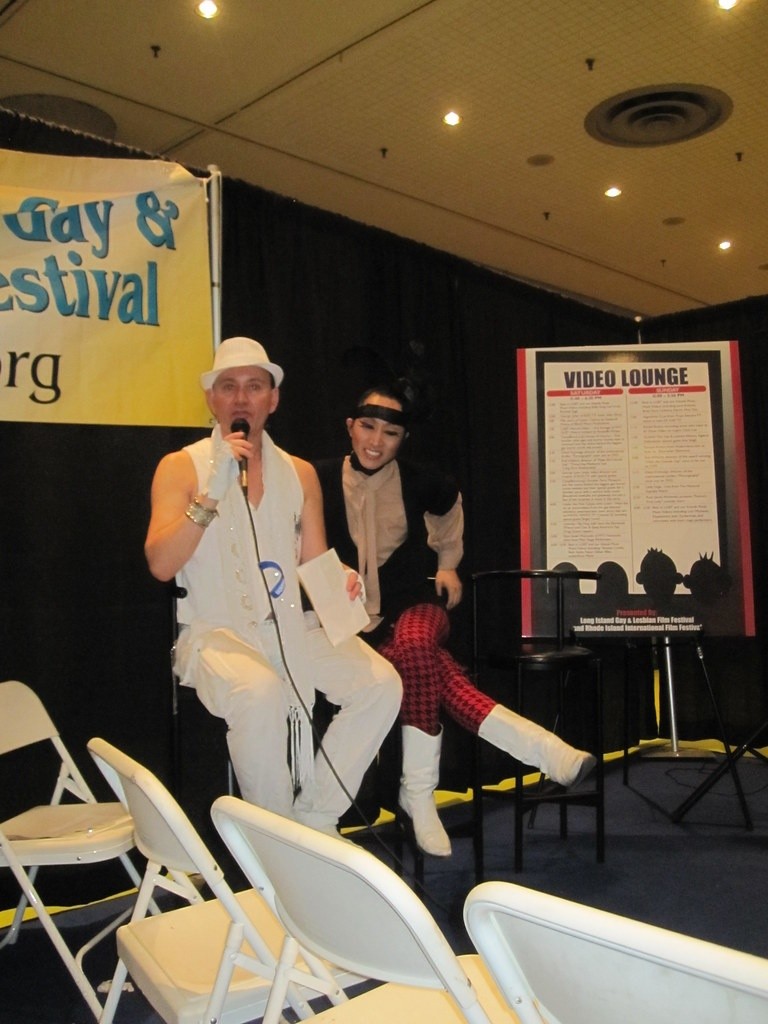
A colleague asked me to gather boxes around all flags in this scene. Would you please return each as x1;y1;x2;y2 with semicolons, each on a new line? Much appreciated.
0;149;215;428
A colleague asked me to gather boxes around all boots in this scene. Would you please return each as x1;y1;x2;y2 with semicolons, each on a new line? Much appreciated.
396;724;452;859
477;702;597;789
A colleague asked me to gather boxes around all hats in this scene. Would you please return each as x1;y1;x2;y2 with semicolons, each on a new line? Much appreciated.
199;336;283;390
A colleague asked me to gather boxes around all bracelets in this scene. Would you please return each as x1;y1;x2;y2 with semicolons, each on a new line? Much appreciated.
186;498;217;529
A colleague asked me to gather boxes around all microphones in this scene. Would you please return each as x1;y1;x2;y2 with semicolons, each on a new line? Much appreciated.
230;417;251;494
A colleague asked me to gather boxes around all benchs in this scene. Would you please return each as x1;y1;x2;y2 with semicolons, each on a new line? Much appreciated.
156;577;487;898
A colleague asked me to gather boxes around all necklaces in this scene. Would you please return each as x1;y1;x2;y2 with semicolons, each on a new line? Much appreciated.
349;452;382;476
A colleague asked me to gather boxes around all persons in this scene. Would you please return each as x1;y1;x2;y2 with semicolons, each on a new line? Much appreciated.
312;382;599;856
142;336;405;843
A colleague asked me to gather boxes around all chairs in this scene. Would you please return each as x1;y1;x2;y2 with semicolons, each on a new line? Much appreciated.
87;737;369;1024
461;882;768;1024
0;681;162;1024
469;568;606;872
212;795;522;1024
